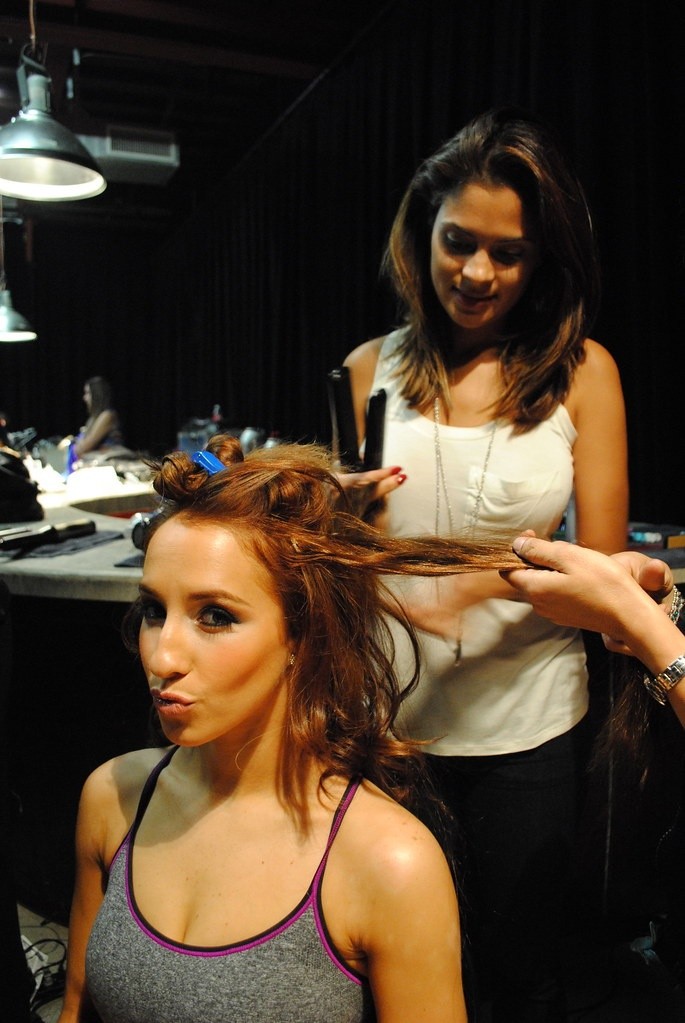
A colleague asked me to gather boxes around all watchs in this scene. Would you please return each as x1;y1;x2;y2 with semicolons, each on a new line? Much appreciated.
642;655;685;706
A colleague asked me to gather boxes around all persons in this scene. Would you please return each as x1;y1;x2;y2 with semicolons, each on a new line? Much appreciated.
502;522;683;1023
317;124;636;1022
52;436;549;1021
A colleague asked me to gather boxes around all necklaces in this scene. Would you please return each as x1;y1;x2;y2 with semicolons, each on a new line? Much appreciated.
420;369;508;670
65;375;124;456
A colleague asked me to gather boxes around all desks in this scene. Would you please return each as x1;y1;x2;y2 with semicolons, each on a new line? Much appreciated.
0;460;685;626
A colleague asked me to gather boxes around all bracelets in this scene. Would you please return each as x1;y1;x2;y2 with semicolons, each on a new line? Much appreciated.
666;584;683;626
